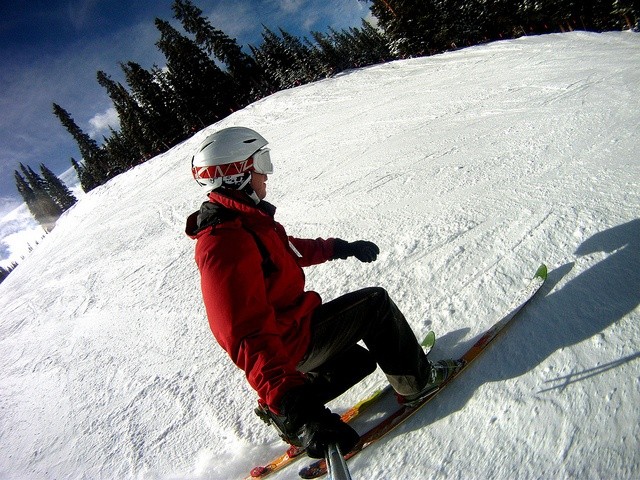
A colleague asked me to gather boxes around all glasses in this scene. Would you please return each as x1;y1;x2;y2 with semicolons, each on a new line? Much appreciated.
192;148;273;180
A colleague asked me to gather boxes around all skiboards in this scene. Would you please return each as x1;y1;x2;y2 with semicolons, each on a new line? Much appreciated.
244;264;547;480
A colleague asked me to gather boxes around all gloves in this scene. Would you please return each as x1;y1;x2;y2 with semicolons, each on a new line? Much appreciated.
273;384;361;459
332;237;380;263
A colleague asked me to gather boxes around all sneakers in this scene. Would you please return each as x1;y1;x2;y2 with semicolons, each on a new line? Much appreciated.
392;358;458;407
253;404;297;447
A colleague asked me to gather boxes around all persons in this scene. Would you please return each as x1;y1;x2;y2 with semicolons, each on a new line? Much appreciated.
186;127;459;459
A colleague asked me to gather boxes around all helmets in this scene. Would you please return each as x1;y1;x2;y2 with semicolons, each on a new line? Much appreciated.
190;126;268;197
